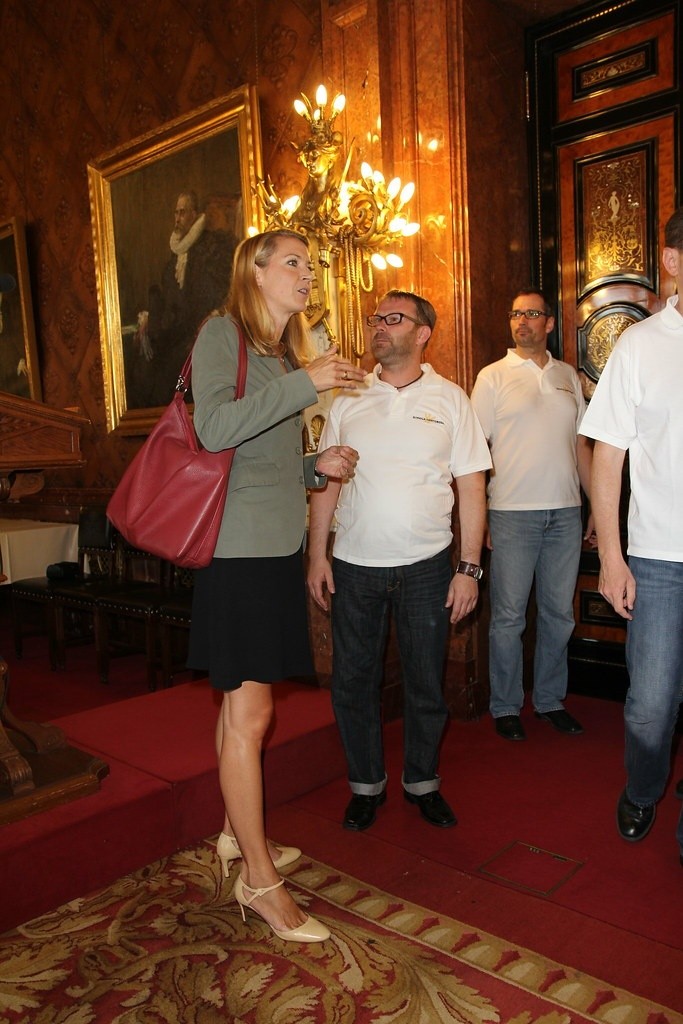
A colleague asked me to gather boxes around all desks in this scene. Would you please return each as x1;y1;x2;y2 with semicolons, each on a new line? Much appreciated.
0;517;80;585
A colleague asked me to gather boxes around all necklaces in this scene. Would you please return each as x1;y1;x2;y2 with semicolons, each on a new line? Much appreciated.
279;357;285;364
379;370;423;390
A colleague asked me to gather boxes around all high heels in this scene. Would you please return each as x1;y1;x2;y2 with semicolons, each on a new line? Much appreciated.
234;873;331;943
217;833;301;878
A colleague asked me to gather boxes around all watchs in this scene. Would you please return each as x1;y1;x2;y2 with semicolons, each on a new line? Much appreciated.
455;560;483;582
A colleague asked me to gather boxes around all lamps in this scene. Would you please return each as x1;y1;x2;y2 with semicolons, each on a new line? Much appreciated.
248;84;419;367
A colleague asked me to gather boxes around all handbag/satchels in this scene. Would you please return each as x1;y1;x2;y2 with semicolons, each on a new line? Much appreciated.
107;318;247;568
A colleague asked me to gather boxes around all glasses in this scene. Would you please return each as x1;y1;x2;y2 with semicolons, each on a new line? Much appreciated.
367;313;423;327
505;310;553;319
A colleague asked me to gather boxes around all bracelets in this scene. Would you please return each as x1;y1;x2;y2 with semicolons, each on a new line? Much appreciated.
314;455;326;477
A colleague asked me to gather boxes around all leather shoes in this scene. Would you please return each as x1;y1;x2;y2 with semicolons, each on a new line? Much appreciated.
496;714;526;740
343;786;388;830
404;788;458;826
616;788;656;841
535;709;585;734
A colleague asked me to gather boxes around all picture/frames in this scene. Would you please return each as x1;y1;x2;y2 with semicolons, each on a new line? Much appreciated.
86;84;266;436
0;216;42;402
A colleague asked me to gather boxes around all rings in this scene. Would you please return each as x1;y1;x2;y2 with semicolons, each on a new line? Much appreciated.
344;371;348;380
347;469;348;472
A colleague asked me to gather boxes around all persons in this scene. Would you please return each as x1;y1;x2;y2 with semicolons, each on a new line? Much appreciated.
186;230;368;942
578;208;683;843
308;290;494;830
469;291;598;741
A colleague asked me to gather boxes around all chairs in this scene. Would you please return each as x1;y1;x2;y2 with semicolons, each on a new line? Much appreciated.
9;507;195;691
